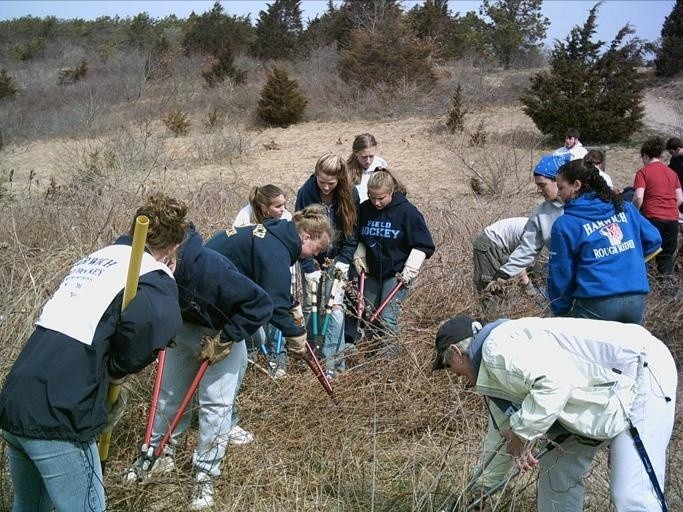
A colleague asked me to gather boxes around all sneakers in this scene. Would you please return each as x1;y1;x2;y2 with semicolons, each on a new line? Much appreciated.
228;426;252;445
123;456;176;481
189;472;215;512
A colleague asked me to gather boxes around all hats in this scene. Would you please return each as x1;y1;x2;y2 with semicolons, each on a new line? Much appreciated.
431;316;483;372
288;300;305;329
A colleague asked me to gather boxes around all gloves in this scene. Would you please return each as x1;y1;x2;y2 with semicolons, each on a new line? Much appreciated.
284;329;307;362
103;388;130;437
193;330;235;367
351;257;368;280
486;270;511;296
394;266;419;289
332;261;350;278
304;267;322;287
521;278;536;299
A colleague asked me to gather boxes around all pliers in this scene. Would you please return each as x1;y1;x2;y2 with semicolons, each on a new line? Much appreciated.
451;426;568;512
354;272;403;346
301;343;347;419
135;346;209;482
308;275;340;360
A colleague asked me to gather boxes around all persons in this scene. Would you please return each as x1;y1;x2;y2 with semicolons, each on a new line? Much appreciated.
472;127;683;327
122;222;275;512
1;192;187;512
203;133;434;445
430;315;678;512
